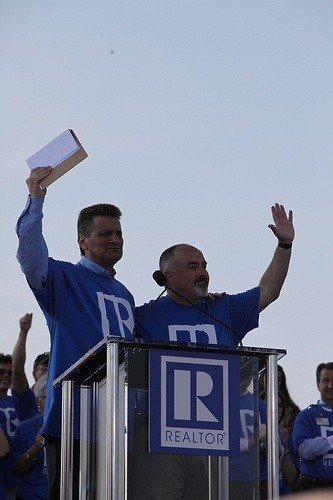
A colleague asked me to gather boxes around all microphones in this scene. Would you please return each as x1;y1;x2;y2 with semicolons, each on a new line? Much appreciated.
152;271;244;348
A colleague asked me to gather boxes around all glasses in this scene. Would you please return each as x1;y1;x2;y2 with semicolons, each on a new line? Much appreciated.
0;368;12;375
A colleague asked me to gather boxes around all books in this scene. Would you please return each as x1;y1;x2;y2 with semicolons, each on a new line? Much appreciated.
26;129;88;190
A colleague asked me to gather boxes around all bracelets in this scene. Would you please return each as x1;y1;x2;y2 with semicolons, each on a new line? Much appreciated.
278;243;292;249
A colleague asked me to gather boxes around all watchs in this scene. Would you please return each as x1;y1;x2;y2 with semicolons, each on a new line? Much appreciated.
25;451;31;462
258;440;264;450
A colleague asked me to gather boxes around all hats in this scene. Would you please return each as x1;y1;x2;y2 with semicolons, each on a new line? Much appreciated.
34;352;49;367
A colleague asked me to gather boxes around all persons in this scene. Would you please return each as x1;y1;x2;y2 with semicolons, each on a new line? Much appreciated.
228;375;284;500
0;423;13;500
11;313;50;422
292;361;333;490
0;352;19;441
136;203;294;500
258;366;301;485
15;165;135;500
14;374;49;500
